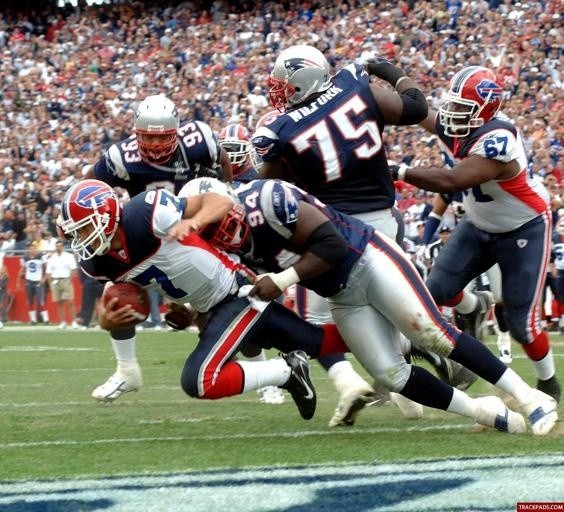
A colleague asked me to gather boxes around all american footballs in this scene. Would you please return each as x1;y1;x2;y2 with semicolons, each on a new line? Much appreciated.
103;281;151;325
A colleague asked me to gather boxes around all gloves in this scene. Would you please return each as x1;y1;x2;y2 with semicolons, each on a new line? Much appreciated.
416;244;430;266
385;161;406;182
367;56;405;84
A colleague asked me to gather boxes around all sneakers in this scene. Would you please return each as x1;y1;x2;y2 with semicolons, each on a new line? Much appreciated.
414;342;477;392
258;385;283;404
389;392;425;420
92;367;144;400
494;325;513;360
328;379;376;429
529;386;558;436
538;378;561;404
457;290;493;345
481;395;527;436
283;349;316;420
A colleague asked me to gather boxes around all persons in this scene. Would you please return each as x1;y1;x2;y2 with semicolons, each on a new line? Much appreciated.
435;0;498;89
56;178;453;421
215;124;257;184
79;95;233;401
506;113;564;212
46;240;80;328
369;0;434;267
2;1;103;244
497;3;564;111
389;63;560;399
252;43;428;430
104;3;249;138
17;243;48;326
176;175;560;436
541;210;564;327
248;0;369;119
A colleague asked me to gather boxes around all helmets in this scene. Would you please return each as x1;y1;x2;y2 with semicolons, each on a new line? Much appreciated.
59;179;121;260
439;64;503;137
265;45;330;112
218;123;257;171
178;176;248;257
134;94;178;165
